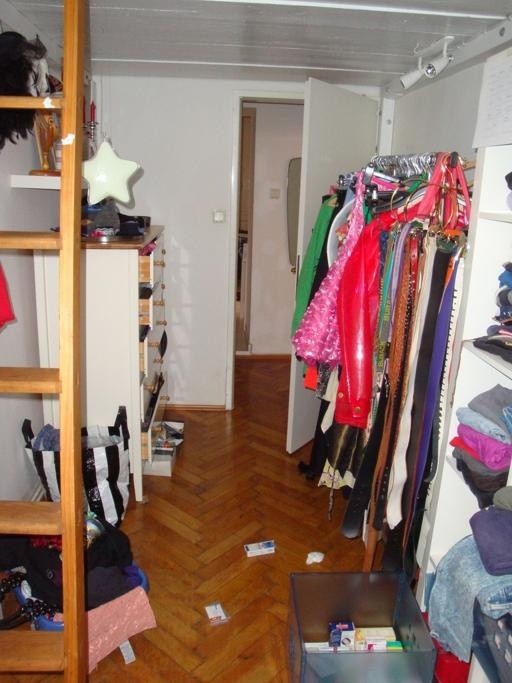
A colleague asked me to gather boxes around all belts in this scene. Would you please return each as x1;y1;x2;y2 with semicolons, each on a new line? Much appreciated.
341;222;466;580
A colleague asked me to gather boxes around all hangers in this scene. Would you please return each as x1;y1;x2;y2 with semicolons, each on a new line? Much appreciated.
336;153;464;213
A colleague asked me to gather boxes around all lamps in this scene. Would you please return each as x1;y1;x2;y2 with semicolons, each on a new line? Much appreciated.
397;35;455;91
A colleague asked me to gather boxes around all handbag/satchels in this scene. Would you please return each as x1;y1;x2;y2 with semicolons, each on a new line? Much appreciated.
0;556;122;630
22;406;130;528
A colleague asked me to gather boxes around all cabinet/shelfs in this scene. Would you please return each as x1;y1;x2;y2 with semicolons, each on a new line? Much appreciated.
0;0;87;682
30;223;185;508
407;142;512;683
